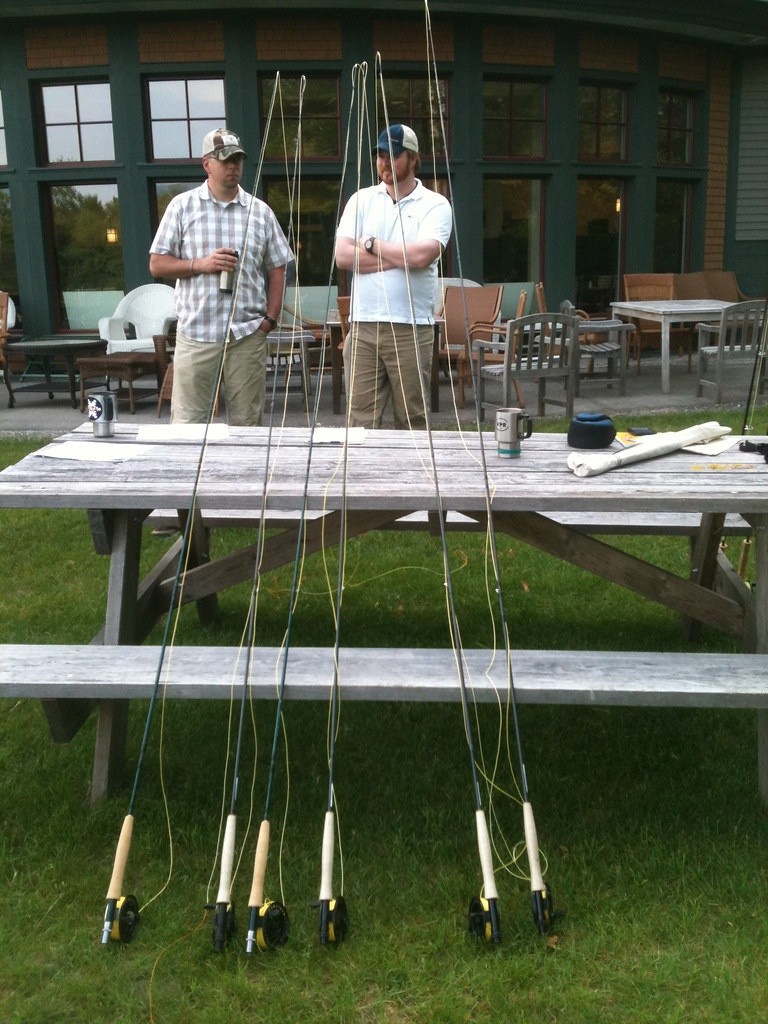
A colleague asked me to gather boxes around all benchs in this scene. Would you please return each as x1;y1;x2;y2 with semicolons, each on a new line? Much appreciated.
0;641;768;709
623;271;754;376
145;508;752;626
436;278;502;354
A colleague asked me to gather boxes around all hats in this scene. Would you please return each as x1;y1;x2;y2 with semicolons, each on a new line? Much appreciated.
371;124;418;155
202;128;248;161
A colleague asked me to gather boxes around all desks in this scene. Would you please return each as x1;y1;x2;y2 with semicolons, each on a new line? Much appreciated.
4;339;111;409
75;351;158;414
609;298;768;394
0;423;768;804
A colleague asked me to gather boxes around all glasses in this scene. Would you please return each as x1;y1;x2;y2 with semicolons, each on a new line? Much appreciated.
207;153;242;164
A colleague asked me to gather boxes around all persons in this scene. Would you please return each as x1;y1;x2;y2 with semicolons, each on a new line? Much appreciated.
332;125;452;429
148;129;295;425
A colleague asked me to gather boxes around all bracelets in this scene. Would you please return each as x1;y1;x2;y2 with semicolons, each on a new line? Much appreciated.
190;259;194;274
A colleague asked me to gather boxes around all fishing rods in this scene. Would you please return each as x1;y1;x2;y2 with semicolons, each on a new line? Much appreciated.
98;2;558;955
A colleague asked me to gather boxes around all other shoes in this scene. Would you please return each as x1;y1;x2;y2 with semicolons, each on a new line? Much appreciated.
151;527;177;537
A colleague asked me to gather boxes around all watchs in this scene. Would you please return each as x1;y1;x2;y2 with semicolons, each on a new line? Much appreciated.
264;316;277;330
365;237;376;255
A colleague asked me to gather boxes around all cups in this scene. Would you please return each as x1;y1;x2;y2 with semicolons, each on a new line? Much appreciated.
495;407;533;459
219;251;239;293
88;391;116;437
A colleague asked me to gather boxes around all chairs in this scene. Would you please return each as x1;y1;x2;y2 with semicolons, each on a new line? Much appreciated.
272;303;331;348
560;300;636;390
482;279;535;321
436;285;503;408
533;281;597;383
326;308;342;414
694;300;768;403
456;289;526;408
266;331;316;413
471;312;580;422
337;296;351;350
98;283;174;353
